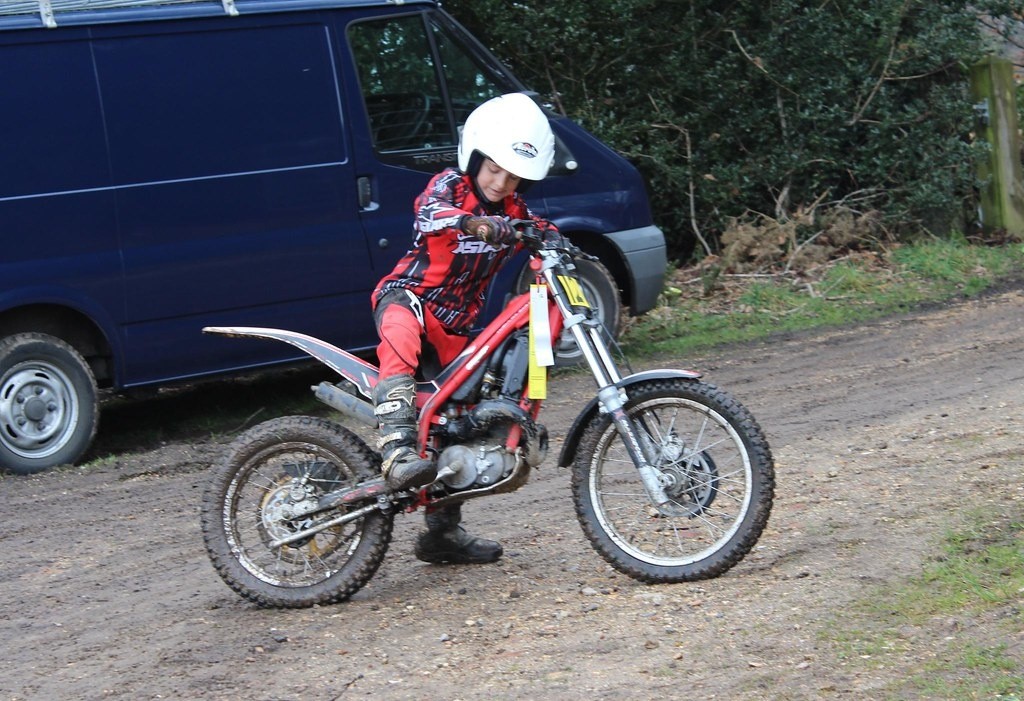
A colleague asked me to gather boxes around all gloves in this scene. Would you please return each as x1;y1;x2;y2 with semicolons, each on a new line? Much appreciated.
542;229;574;249
463;215;516;244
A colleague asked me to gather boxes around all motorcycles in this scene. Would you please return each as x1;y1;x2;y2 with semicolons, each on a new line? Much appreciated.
199;218;777;609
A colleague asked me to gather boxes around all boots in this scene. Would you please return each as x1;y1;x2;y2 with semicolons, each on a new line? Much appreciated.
415;501;503;563
371;375;438;495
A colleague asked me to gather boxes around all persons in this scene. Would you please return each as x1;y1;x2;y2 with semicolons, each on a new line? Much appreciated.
371;92;558;565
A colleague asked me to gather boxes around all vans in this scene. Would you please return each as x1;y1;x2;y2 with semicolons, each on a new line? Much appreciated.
0;0;670;475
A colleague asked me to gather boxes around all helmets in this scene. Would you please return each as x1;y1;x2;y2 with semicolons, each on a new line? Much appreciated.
458;93;555;193
